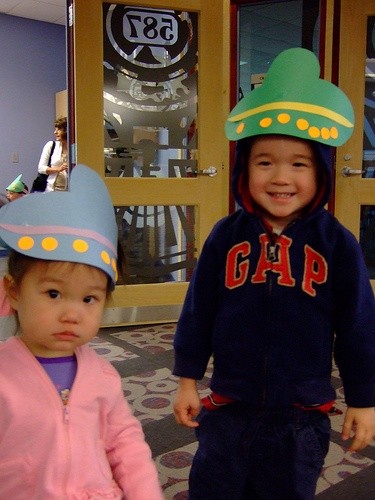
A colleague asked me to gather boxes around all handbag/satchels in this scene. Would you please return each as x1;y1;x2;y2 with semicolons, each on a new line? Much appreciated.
29;169;50;193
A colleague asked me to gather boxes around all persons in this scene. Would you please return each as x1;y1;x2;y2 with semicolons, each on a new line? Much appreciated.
0;164;165;500
31;116;68;194
173;49;375;500
6;174;29;202
